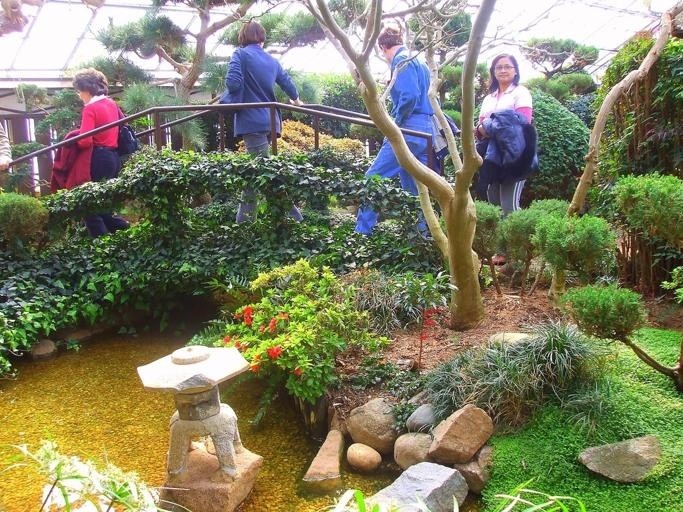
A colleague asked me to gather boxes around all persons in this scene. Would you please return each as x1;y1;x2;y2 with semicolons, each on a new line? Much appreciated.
0;123;13;171
341;22;436;237
207;16;308;226
472;50;533;265
66;67;137;239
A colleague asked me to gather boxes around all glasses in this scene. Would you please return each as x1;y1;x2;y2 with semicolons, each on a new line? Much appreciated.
494;65;516;71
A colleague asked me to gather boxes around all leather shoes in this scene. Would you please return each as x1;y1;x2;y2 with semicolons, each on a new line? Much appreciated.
479;253;509;266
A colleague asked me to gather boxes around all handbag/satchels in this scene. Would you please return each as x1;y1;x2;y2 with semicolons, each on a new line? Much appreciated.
118;124;138;156
